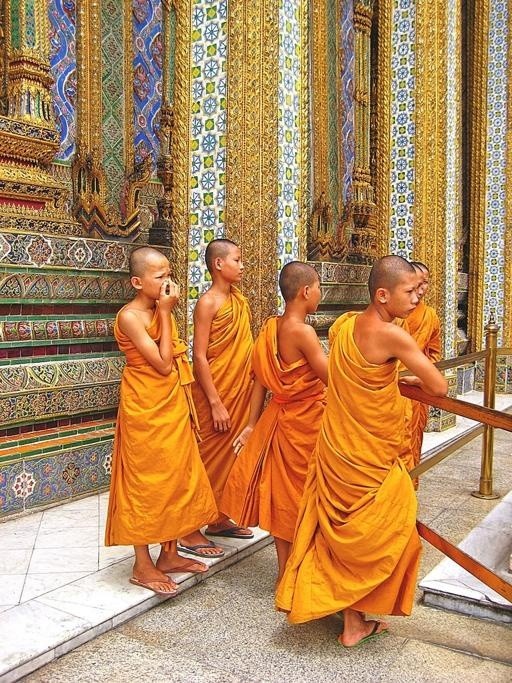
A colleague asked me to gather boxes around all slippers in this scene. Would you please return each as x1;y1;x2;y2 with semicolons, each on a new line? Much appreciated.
164;558;209;572
205;520;254;538
177;542;224;557
130;575;177;595
338;620;389;648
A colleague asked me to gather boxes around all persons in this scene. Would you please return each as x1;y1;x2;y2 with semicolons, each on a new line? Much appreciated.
216;261;332;590
176;237;253;557
102;245;209;596
402;262;442;491
410;263;423;302
274;252;448;648
